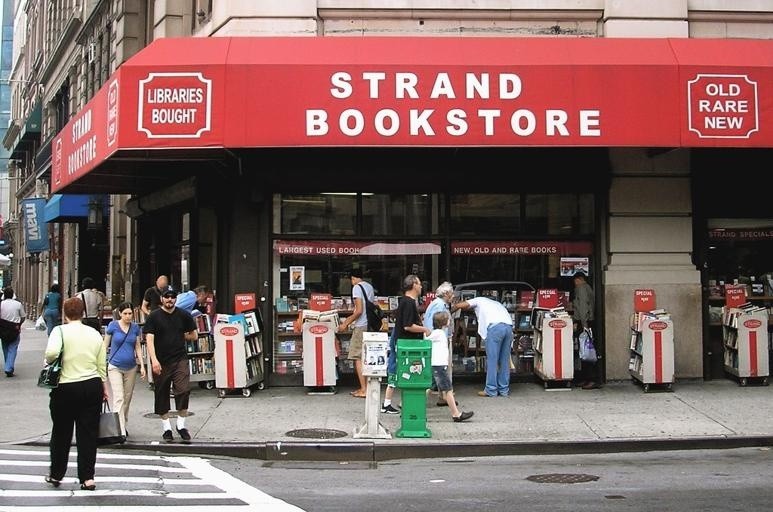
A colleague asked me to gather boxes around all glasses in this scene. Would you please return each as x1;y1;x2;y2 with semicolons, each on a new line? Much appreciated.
165;295;176;298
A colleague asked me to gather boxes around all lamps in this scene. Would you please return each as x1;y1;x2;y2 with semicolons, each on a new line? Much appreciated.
80;198;113;249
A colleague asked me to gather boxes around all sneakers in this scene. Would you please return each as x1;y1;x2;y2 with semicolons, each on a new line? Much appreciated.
478;391;490;396
4;370;13;377
574;381;601;389
437;401;457;406
163;425;190;441
381;404;400;414
351;389;366;398
452;412;474;422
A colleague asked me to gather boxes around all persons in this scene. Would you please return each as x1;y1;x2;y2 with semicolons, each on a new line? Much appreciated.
33;271;212;445
34;296;112;492
570;271;604;391
0;287;26;378
338;270;384;399
421;280;461;407
417;311;475;424
373;274;432;416
449;296;516;398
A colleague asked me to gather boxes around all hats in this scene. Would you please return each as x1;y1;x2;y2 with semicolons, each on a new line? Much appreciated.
161;285;178;296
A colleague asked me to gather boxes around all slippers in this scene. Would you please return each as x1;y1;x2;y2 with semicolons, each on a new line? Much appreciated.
45;477;61;488
81;483;96;491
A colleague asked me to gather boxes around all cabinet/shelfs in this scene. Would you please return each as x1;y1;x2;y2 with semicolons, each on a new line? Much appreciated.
708;295;773;387
628;310;676;393
98;289;581;397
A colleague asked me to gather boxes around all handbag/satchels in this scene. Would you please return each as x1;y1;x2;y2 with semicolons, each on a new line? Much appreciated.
37;366;61;389
96;398;122;447
578;332;602;362
366;301;383;332
45;296;48;305
0;319;21;341
87;321;98;329
